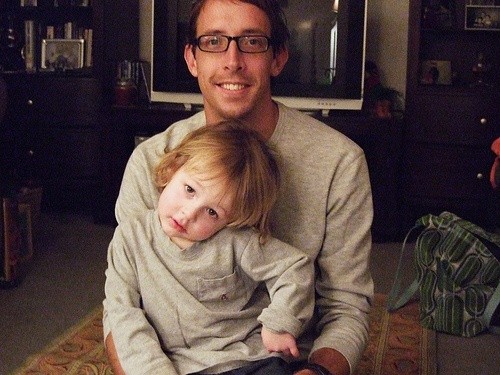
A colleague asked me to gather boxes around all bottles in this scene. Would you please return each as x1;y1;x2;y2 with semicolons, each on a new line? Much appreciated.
115;80;138;106
471;51;488;87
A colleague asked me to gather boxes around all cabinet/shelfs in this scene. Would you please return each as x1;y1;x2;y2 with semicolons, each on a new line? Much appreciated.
93;104;403;243
0;0;105;212
400;0;500;230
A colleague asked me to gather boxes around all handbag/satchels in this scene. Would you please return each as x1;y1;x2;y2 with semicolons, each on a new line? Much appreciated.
385;211;500;338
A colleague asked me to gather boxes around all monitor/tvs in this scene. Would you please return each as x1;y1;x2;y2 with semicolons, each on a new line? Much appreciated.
151;0;368;118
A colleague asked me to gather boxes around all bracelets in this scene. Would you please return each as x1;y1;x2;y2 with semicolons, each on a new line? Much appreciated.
304;363;331;375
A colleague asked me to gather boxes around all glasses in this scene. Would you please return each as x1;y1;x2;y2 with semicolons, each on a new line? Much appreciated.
192;34;273;54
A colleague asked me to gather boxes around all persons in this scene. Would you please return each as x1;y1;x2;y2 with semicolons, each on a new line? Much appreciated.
103;0;374;375
105;120;315;375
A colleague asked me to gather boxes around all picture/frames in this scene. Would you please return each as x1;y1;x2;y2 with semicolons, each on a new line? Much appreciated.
464;5;500;30
41;39;84;70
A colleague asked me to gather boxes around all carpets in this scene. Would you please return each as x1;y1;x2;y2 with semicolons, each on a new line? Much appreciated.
9;290;438;375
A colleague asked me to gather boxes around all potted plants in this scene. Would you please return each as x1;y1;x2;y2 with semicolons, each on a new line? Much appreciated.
369;85;400;119
